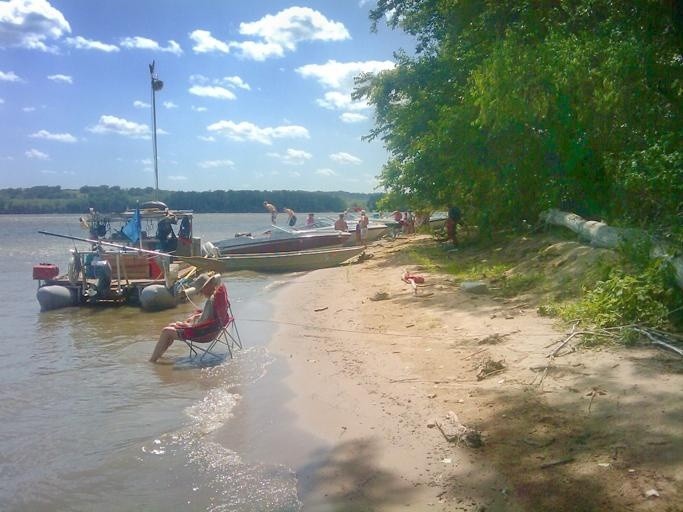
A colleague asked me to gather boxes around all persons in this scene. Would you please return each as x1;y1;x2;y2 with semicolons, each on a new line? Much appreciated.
262;200;278;226
303;213;314;226
354;220;361;246
406;211;415;233
333;213;348;233
390;208;402;238
401;212;408;234
359;210;368;249
150;272;221;362
282;207;297;226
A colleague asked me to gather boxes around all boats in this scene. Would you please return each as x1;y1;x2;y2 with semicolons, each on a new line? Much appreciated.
34;59;216;315
170;208;449;273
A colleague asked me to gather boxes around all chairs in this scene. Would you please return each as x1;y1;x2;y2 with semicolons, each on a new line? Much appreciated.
185;283;243;361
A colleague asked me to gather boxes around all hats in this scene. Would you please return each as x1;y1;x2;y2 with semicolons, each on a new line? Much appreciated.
192;273;215;294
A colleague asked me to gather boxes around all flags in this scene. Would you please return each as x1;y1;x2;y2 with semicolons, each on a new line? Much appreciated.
120;206;140;243
